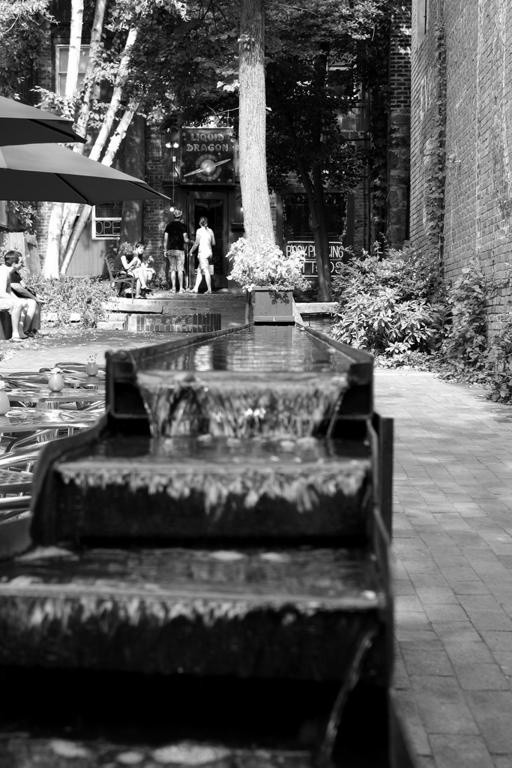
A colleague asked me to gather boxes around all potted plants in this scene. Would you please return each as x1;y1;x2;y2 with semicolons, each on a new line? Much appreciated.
224;237;314;324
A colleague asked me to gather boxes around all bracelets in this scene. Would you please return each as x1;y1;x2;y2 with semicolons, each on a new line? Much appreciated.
146;261;150;265
162;249;167;252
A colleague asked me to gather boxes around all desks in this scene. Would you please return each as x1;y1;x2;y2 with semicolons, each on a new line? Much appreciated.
0;362;107;513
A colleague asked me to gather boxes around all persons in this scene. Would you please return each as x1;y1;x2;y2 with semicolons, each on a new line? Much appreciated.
0;249;29;344
132;240;156;297
188;216;217;295
8;263;46;339
162;208;194;294
112;239;151;299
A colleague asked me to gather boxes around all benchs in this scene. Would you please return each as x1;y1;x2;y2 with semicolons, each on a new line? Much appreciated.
104;253;137;300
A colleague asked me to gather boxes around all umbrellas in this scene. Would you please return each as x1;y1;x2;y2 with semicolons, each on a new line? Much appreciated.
0;93;90;151
0;140;173;204
